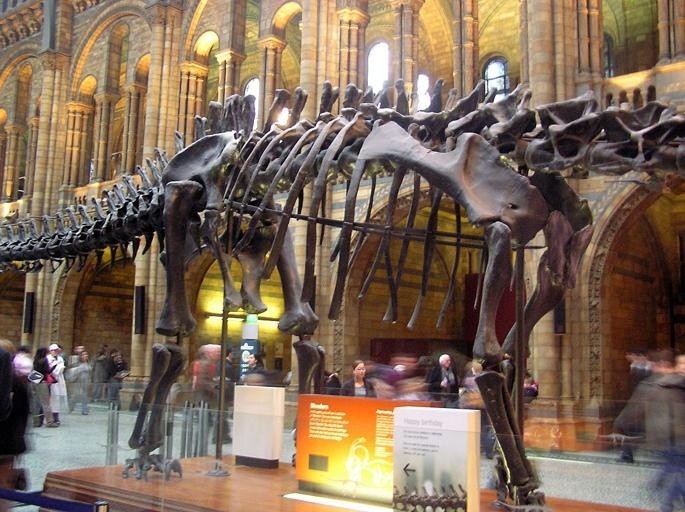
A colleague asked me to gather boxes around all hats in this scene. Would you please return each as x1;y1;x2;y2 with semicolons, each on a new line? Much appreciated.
48;343;62;352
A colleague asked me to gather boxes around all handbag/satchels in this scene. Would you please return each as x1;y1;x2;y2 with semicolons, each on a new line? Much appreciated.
27;369;44;385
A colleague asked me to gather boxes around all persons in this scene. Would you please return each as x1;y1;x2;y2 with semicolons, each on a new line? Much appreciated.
613;347;685;512
0;340;539;512
